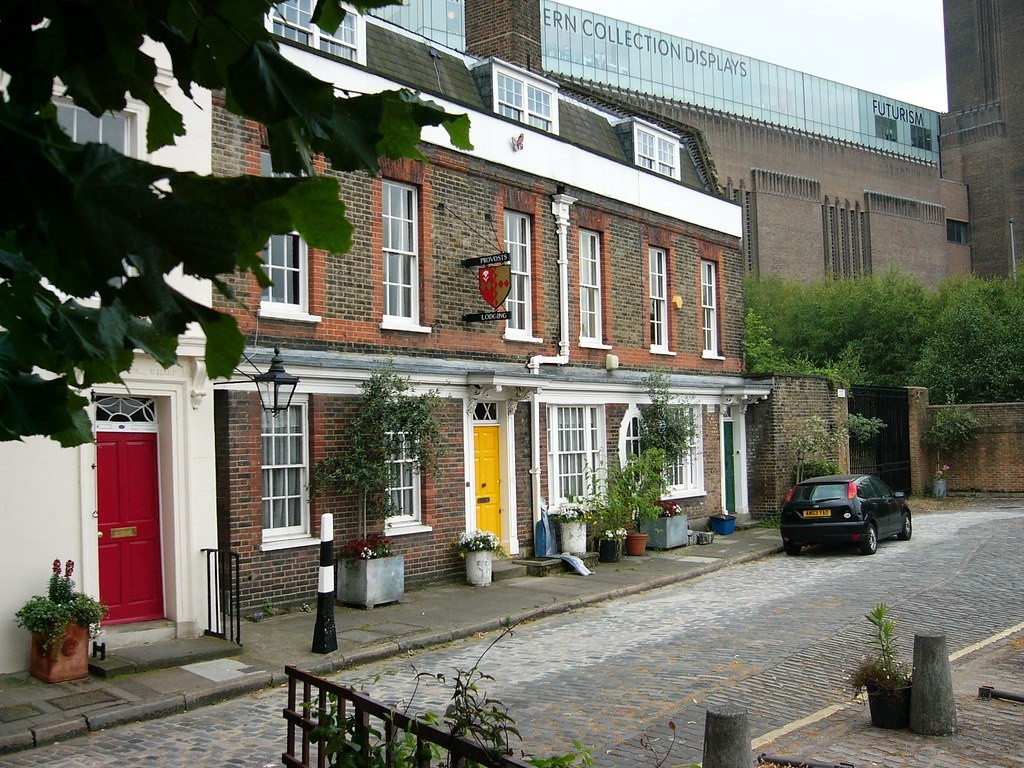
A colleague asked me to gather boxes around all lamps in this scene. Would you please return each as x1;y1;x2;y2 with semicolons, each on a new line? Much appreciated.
214;348;299;420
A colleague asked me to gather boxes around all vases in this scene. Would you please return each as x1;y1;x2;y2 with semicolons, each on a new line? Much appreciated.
29;621;90;683
931;479;947;498
465;550;492;586
560;521;586;555
336;555;405;610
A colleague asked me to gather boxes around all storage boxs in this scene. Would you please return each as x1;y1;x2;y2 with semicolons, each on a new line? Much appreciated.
708;514;735;534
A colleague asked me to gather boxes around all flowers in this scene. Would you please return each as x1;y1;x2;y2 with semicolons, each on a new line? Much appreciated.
936;464;949;480
549;503;593;523
341;532;399;561
446;528;513;560
15;559;104;629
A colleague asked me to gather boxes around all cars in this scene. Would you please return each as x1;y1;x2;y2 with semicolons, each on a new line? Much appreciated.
780;474;912;555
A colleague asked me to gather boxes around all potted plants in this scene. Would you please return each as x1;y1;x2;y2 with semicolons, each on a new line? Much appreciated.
621;447;671;556
639;362;688;548
564;450;638;562
847;603;913;729
